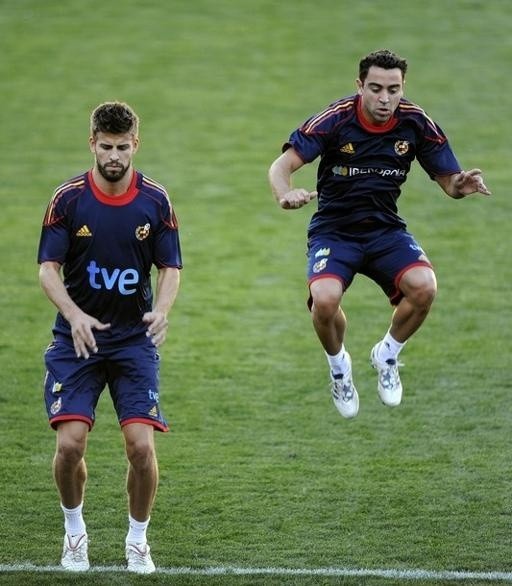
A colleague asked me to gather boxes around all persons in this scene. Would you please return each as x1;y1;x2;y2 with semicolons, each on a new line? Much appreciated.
36;99;184;576
268;46;492;422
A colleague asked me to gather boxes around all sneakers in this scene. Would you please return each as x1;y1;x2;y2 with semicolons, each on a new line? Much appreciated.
329;352;359;418
370;340;406;407
61;532;90;572
125;538;156;575
